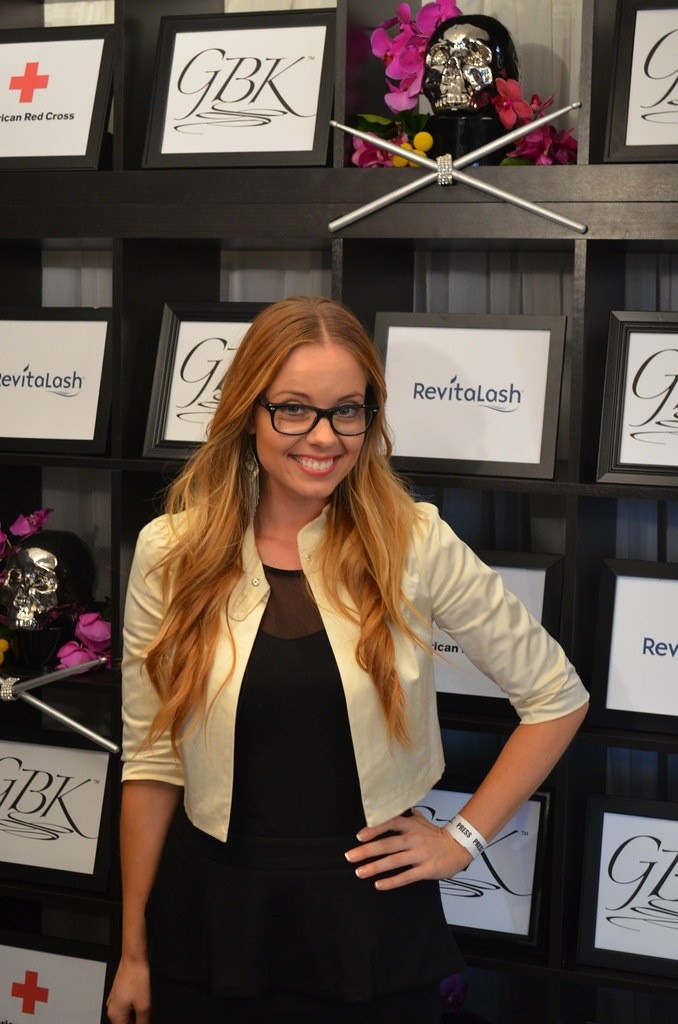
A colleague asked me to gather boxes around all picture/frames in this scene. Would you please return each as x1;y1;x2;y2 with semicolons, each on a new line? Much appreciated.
431;548;565;718
590;558;678;736
0;24;114;171
142;301;279;460
141;7;337;168
575;792;678;979
0;933;114;1024
602;0;678;164
371;310;568;480
0;730;119;889
411;785;552;948
0;306;112;454
577;310;678;487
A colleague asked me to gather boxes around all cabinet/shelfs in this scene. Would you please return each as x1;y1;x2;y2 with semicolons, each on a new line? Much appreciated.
0;0;678;1024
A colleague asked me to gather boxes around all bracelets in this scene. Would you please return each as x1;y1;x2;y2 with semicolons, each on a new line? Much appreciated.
444;812;488;861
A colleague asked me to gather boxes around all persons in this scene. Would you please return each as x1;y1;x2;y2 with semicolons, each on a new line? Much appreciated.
106;296;591;1024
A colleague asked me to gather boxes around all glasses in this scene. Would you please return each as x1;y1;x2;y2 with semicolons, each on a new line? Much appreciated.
254;394;379;436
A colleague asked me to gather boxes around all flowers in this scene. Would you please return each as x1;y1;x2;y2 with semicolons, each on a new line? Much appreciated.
55;613;111;673
492;77;577;166
351;110;433;168
370;0;463;115
0;508;55;556
